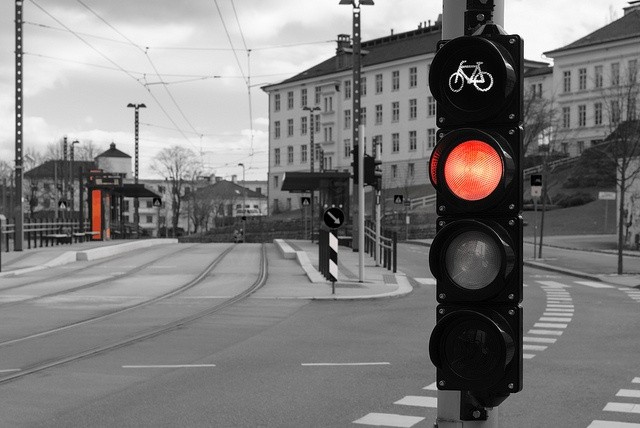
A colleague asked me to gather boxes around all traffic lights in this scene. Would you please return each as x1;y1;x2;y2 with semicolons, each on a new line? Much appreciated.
351;145;358;184
365;157;382;184
429;24;523;408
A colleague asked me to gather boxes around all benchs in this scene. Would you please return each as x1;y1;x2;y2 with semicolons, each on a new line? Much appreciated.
85;231;101;242
72;233;85;242
41;234;71;244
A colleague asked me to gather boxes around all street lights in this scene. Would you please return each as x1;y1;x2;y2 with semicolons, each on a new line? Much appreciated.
339;0;374;251
238;163;245;244
71;141;79;220
128;103;147;236
303;107;320;242
